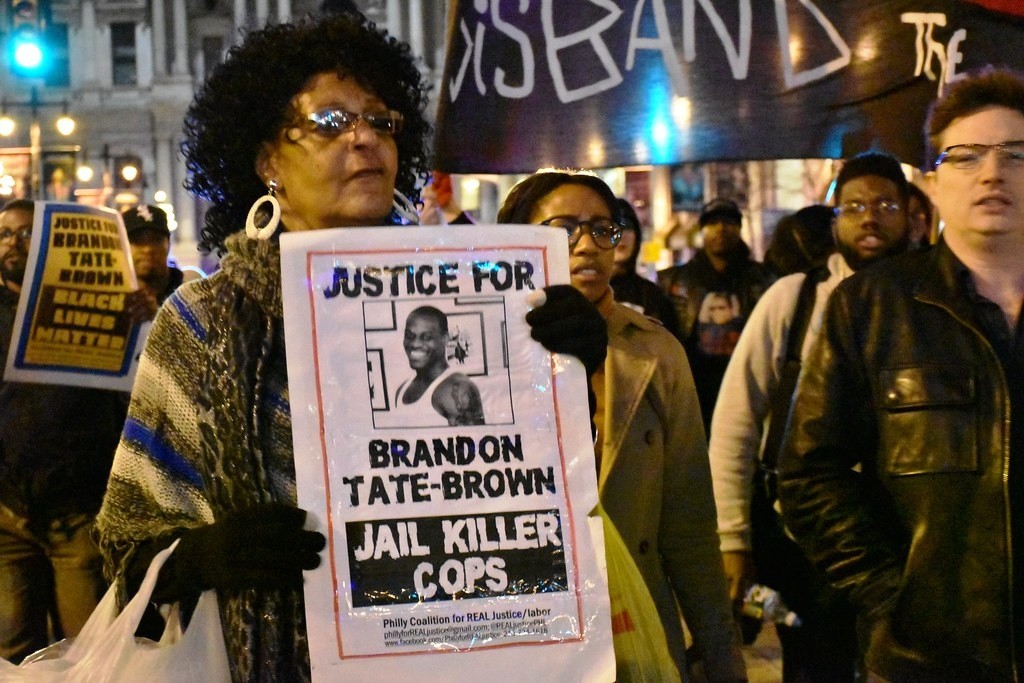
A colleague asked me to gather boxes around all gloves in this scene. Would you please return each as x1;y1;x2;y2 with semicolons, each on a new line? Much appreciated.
125;503;324;600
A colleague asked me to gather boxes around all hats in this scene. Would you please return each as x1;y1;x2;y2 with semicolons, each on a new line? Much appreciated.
700;197;741;227
122;206;170;238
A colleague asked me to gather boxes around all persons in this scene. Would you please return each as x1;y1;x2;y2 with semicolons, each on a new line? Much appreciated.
0;149;932;683
392;306;487;425
96;13;609;683
777;73;1024;683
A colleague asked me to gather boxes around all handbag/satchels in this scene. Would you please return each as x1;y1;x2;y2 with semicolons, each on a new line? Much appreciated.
1;534;236;683
744;459;783;591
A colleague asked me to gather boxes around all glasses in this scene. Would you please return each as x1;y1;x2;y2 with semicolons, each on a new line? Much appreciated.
834;200;905;214
291;106;403;137
539;215;626;250
1;228;28;244
908;209;926;219
935;142;1023;169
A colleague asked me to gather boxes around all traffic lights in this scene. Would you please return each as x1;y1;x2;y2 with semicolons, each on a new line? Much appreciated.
9;0;46;78
